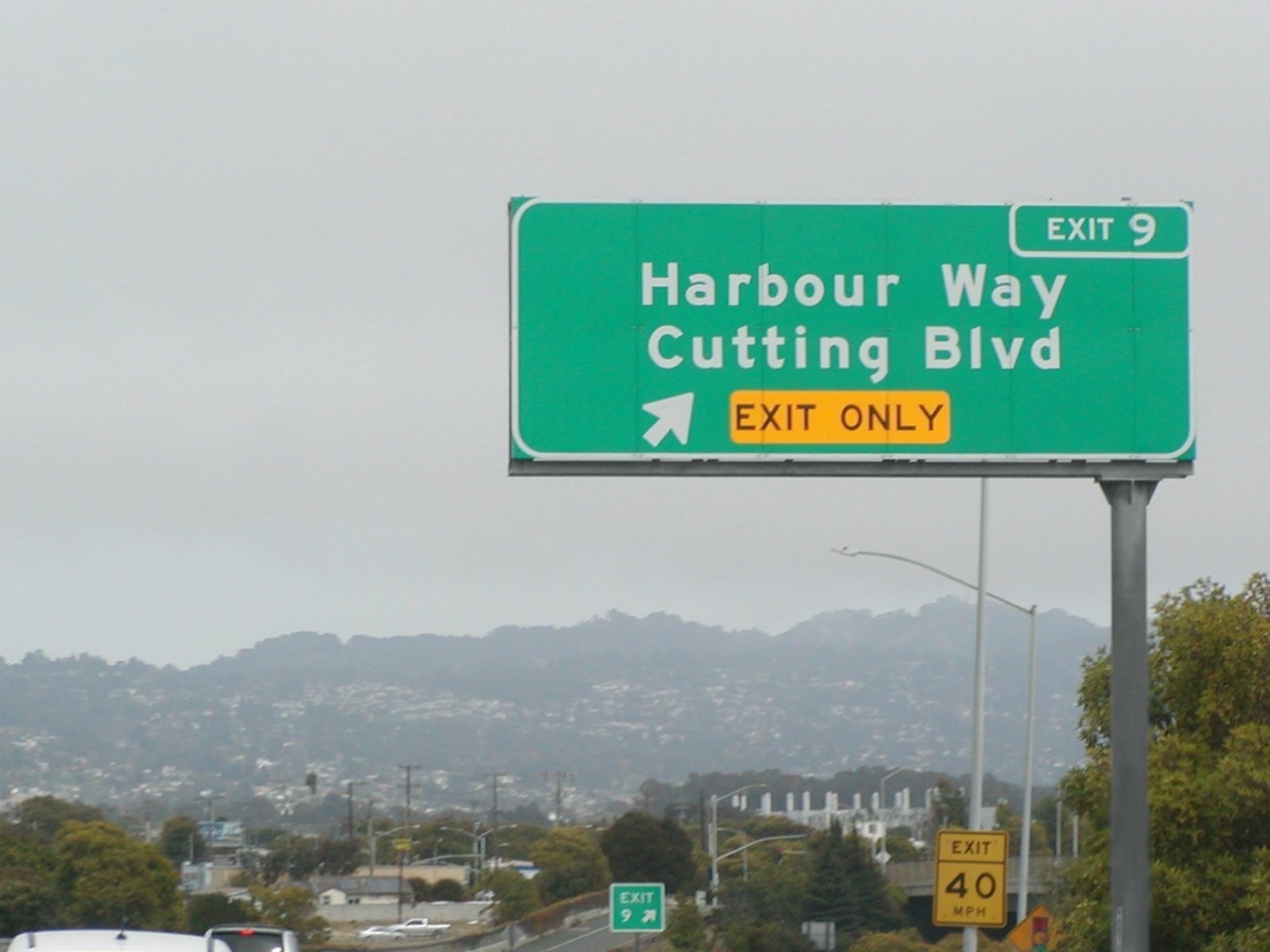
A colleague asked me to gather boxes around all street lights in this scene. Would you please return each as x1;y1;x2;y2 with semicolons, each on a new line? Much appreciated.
473;823;519;887
369;824;420;877
440;825;486;869
831;544;1038;925
711;783;767;904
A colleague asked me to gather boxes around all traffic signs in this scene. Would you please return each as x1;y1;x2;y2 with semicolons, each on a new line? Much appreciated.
507;196;1198;464
609;883;665;933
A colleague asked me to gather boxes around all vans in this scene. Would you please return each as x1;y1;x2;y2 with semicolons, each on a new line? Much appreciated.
6;920;302;952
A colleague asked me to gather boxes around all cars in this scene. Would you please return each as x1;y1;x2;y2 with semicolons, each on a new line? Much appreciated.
354;924;406;943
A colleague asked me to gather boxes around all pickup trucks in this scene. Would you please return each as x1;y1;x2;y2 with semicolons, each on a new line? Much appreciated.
387;917;452;940
